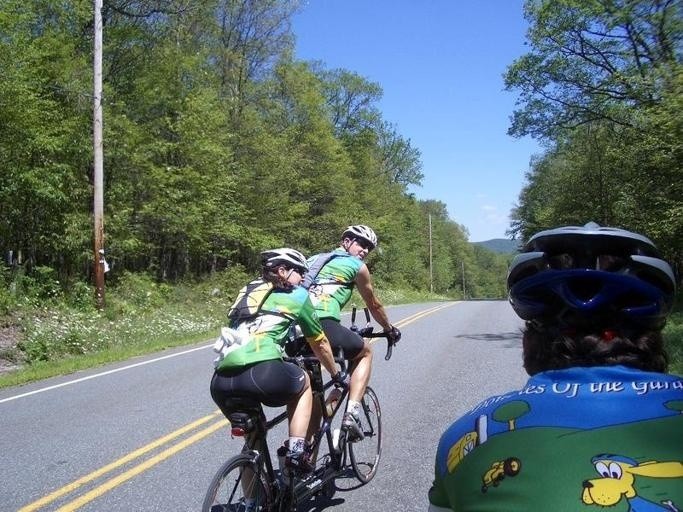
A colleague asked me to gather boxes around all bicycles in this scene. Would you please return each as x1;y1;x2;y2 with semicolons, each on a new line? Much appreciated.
200;306;393;511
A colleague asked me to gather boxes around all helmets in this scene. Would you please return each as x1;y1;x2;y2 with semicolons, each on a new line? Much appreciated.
260;247;309;273
341;224;379;247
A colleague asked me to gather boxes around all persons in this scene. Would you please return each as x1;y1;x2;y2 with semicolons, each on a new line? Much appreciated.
297;224;402;468
424;219;683;512
206;244;351;510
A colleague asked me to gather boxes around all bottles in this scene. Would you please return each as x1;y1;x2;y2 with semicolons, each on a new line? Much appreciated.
276;445;291;485
324;389;342;415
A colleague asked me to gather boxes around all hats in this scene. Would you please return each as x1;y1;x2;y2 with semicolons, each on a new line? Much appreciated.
505;221;678;319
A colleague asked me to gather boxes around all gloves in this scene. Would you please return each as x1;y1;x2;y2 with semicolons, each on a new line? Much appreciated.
385;325;403;345
333;370;352;387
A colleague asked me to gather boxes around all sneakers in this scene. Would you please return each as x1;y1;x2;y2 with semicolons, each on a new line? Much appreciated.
243;478;279;511
341;413;365;443
283;449;315;473
